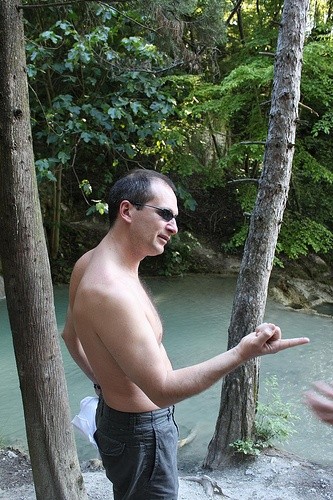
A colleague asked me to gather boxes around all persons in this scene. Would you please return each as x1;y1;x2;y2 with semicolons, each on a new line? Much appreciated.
61;170;310;500
304;381;333;426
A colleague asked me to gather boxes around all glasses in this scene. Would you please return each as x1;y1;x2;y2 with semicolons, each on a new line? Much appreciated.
132;203;183;226
